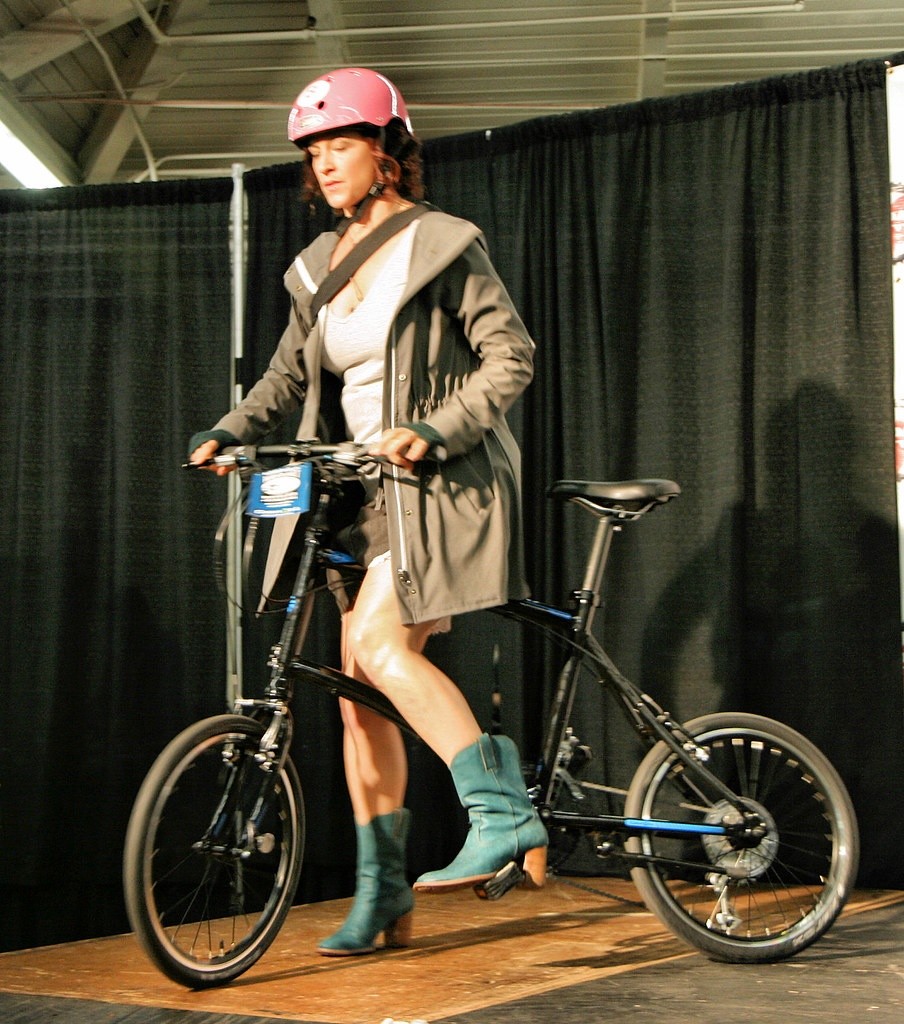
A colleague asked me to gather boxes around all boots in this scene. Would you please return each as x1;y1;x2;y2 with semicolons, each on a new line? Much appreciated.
315;808;415;956
412;733;549;895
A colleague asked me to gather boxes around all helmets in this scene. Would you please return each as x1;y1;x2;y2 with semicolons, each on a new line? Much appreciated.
287;68;414;142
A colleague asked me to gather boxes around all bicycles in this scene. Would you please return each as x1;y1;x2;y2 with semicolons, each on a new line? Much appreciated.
122;438;862;987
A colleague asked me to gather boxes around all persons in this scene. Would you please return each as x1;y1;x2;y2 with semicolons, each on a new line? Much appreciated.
189;68;549;955
